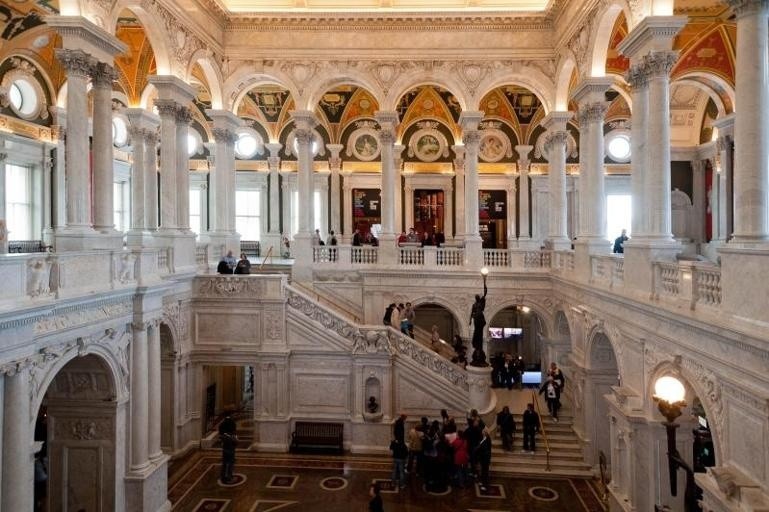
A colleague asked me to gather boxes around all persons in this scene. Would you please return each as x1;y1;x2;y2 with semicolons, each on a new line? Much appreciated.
367;484;384;511
490;351;525;391
496;406;516;451
284;228;444;264
451;334;468;371
389;408;492;493
429;324;441;353
520;403;540;455
469;276;489;367
217;249;250;274
614;230;629;253
383;303;415;339
219;412;237;484
539;362;565;422
572;237;577;249
368;396;378;413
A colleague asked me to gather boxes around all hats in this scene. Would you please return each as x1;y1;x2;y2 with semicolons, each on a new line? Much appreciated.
398;302;406;309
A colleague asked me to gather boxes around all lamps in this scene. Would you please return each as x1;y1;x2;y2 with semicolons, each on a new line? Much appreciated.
481;266;491;299
651;371;701;498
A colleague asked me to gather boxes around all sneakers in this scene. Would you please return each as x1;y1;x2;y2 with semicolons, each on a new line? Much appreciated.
530;450;535;456
520;449;528;453
547;402;561;422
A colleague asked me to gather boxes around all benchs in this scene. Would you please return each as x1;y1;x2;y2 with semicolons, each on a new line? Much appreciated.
242;242;259;256
8;239;55;253
289;421;342;451
